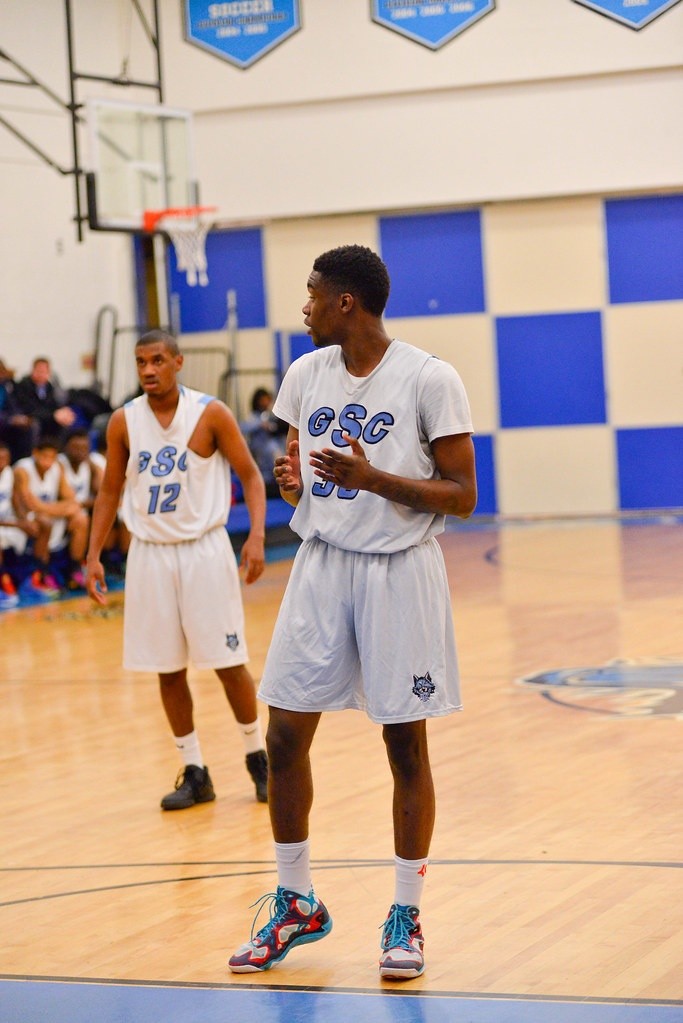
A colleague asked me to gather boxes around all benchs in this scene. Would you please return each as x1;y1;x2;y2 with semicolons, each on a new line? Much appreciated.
0;473;297;592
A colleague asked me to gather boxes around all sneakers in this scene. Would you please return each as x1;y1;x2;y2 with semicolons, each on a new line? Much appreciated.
161;765;215;809
378;904;425;979
246;750;269;801
228;884;332;973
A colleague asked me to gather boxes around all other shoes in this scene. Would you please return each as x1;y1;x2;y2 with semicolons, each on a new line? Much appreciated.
70;572;86;589
30;571;62;598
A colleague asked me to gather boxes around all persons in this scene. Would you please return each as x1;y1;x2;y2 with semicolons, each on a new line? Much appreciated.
235;387;292;501
0;357;134;612
226;244;477;980
81;330;270;813
